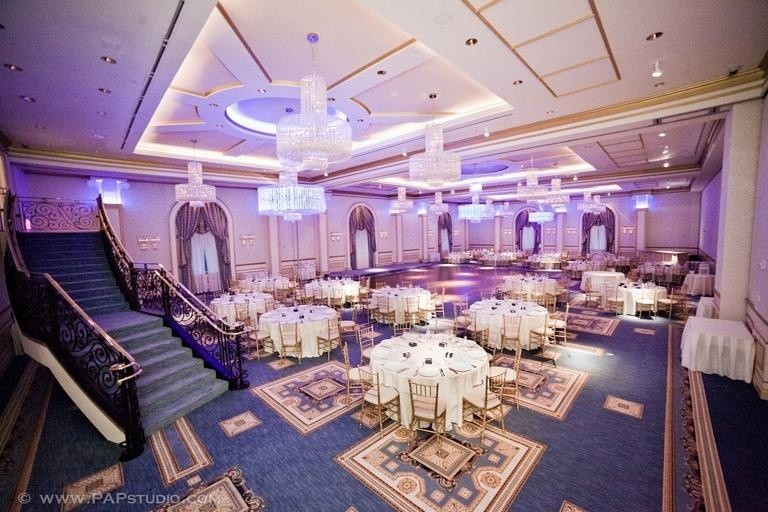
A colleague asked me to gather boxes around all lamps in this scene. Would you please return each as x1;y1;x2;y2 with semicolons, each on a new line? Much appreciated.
175;33;607;222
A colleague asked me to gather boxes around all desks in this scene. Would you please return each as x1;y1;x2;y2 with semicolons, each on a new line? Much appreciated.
681;273;715;296
695;296;714;318
680;316;756;384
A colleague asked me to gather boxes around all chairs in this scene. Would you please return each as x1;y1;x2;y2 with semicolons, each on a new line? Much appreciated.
203;248;687;456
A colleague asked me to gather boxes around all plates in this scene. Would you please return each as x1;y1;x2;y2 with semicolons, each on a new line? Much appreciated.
473;299;546;318
261;304;337;322
373;333;486;377
